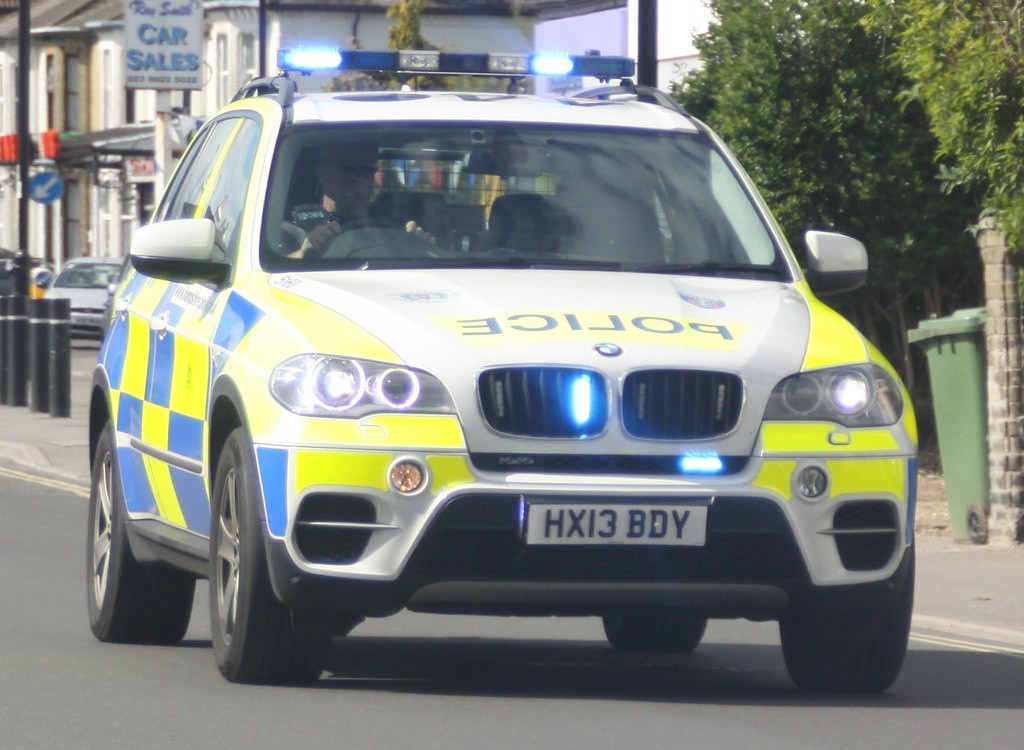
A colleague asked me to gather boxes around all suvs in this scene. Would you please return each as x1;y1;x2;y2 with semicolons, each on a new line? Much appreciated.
84;46;917;693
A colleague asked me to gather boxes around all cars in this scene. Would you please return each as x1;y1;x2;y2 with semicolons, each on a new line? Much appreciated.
30;257;129;345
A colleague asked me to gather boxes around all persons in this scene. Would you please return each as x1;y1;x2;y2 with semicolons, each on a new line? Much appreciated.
287;141;439;259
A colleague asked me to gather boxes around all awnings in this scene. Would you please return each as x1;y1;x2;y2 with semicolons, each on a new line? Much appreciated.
54;121;155;169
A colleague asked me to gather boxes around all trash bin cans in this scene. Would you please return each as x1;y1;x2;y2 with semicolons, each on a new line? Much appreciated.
907;307;988;545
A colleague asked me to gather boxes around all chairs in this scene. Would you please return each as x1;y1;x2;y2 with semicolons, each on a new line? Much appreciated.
368;190;455;245
489;192;556;236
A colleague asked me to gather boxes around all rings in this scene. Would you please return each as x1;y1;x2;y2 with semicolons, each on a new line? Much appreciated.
422;232;430;239
